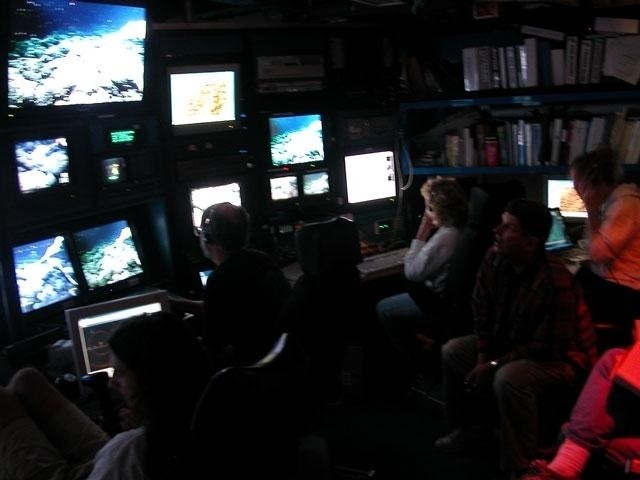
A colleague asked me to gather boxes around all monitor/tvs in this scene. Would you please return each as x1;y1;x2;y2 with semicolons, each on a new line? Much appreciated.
64;289;169;398
74;219;146;301
345;151;396;204
4;234;79;327
545;208;573;250
7;1;147;109
547;179;589;218
270;176;298;200
14;137;70;194
268;114;325;167
190;183;242;236
170;71;236;126
102;158;127;183
199;271;212;286
110;130;135;143
303;172;329;195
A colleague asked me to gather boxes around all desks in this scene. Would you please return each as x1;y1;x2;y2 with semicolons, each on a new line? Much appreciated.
283;246;407;289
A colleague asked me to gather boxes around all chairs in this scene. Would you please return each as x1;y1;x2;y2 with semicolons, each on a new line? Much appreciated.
385;178;526;386
232;218;362;428
186;365;256;480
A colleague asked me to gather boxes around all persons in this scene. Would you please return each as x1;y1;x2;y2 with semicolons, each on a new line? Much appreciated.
572;149;640;319
375;176;486;334
1;312;209;480
521;319;640;480
435;200;596;480
165;202;302;365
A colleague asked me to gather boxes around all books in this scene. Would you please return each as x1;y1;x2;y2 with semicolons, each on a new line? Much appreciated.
443;114;639;167
464;17;640;91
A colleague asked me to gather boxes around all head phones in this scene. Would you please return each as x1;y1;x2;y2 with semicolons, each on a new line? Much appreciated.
200;202;250;245
425;179;455;217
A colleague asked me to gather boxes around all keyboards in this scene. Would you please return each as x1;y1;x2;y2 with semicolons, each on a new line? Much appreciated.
357;248;410;274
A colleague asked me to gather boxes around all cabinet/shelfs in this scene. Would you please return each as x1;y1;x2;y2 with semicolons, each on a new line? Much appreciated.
379;90;640;177
149;0;371;28
